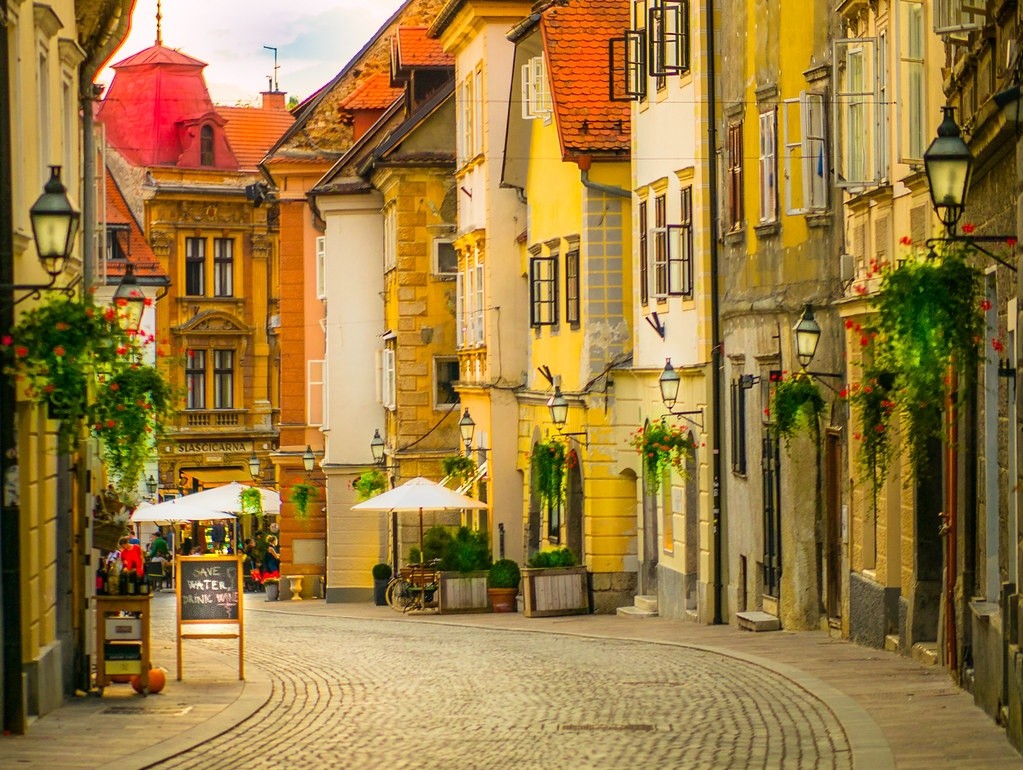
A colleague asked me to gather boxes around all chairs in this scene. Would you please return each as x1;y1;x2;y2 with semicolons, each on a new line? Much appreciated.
143;562;166;592
399;567;436;615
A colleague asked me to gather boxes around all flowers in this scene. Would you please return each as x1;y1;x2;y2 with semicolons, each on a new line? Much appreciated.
345;469;388;504
623;417;707;497
763;370;827;465
838;223;1018;521
524;440;579;514
0;284;194;509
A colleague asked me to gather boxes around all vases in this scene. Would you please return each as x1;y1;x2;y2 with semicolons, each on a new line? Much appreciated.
877;372;910;391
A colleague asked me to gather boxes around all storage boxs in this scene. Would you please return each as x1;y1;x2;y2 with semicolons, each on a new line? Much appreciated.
104;616;142;641
105;653;142;676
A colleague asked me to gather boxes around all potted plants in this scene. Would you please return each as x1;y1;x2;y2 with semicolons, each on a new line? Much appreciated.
372;563;392;606
288;483;318;520
236;485;268;524
95;483;141;519
408;523;494;616
441;455;482;490
487;558;521;613
91;504;133;553
519;547;591;618
264;576;279;601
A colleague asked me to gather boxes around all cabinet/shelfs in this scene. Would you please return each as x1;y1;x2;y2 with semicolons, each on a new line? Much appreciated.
91;592;154;699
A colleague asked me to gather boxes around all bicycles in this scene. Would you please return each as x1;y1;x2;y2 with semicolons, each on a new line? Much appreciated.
385;558;440;614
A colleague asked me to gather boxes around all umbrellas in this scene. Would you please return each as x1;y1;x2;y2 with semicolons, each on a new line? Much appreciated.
129;499;237;591
123;501;192;539
351;471;490;616
173;481;280;556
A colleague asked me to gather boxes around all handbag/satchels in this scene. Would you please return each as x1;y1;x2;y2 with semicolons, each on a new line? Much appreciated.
251;568;261;582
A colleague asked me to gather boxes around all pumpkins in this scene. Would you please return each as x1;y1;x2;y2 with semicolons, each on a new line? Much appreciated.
111;662;165;694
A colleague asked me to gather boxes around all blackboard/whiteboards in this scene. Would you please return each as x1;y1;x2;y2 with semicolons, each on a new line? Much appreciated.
176;554;244;624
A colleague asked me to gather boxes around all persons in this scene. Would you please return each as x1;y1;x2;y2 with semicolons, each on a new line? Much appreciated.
119;537;145;595
151;551;167;588
265;534;280;577
238;547;251;590
149;531;169;559
125;532;140;544
179;537;234;555
244;538;261;593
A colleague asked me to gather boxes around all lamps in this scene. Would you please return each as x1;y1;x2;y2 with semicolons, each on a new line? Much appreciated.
302;445;328;488
244;182;268;209
459;407;492;461
370;428;400;468
112;264;146;336
548;386;588;450
740;374;761;389
143;474;158;500
0;165;81;315
927;106;1017;274
661;357;705;433
792;303;844;393
248;452;279;486
